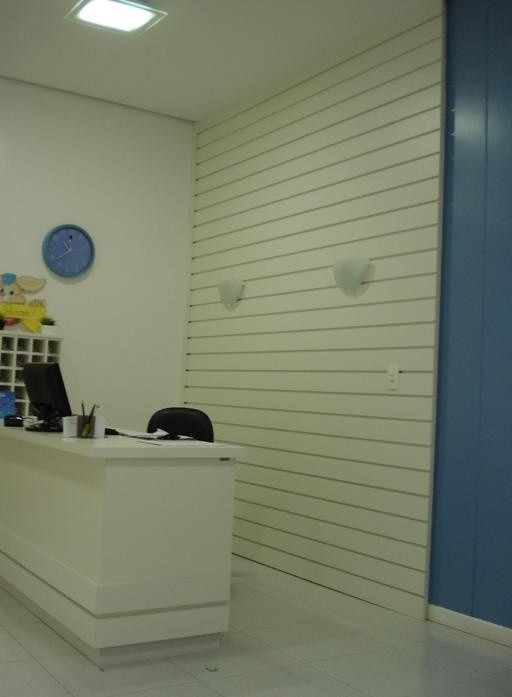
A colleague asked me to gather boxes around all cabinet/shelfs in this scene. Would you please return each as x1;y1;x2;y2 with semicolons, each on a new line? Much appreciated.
0;329;66;416
0;413;243;671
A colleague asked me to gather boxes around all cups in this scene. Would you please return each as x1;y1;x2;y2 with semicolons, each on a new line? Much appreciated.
61;415;107;439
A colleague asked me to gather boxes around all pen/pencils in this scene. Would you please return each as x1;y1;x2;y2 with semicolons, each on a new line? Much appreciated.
81;402;96;436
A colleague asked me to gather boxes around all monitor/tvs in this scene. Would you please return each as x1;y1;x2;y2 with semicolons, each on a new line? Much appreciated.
22;361;72;432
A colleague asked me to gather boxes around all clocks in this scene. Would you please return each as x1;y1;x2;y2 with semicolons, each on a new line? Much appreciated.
42;224;96;278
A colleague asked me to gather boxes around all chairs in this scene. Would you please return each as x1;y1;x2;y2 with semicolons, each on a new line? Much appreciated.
147;407;214;442
